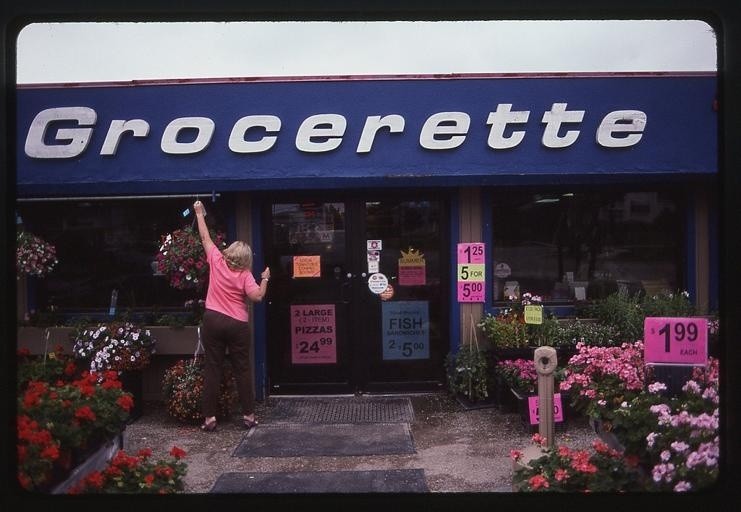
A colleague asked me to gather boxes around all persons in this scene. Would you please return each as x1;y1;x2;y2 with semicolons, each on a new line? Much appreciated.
190;200;273;430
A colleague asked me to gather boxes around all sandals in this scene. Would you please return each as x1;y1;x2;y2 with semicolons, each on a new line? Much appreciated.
243;417;259;427
200;421;216;432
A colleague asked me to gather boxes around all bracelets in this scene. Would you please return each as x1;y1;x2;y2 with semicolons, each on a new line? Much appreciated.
261;277;269;281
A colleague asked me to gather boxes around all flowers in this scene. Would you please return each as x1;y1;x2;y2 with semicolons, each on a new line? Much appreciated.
17;225;228;494
476;287;719;493
